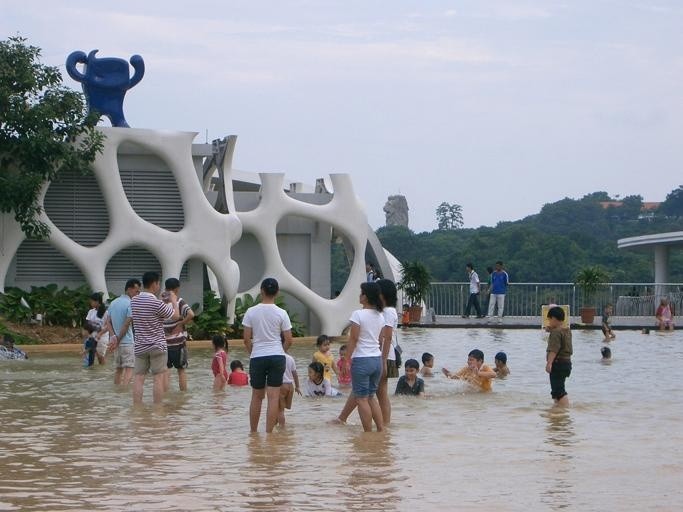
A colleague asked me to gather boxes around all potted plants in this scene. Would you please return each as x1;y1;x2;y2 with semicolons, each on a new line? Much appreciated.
574;264;612;324
394;260;432;323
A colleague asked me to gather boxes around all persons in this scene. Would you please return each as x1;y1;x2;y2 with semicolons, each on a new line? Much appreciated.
246;437;403;512
442;349;508;389
654;296;674;332
480;267;504;317
364;262;381;283
484;259;509;323
84;268;193;399
599;347;612;360
0;333;29;361
540;408;578;511
339;278;399;432
305;333;434;396
601;304;616;342
461;264;484;320
242;278;293;432
542;307;573;410
212;333;302;418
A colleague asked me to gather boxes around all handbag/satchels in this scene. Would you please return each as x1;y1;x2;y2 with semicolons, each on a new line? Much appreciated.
395;345;404;367
